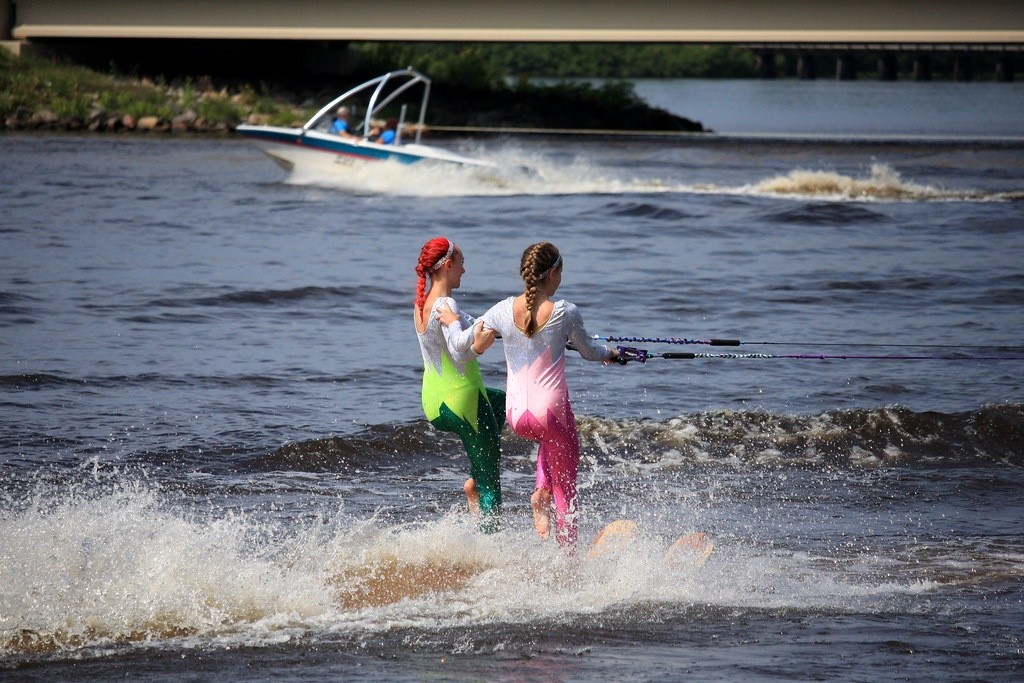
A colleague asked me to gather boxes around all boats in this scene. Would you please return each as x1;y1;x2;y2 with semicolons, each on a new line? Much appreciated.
234;65;497;176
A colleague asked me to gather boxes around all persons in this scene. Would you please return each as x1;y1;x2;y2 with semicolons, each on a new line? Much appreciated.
330;106;396;143
434;242;622;561
414;238;506;535
